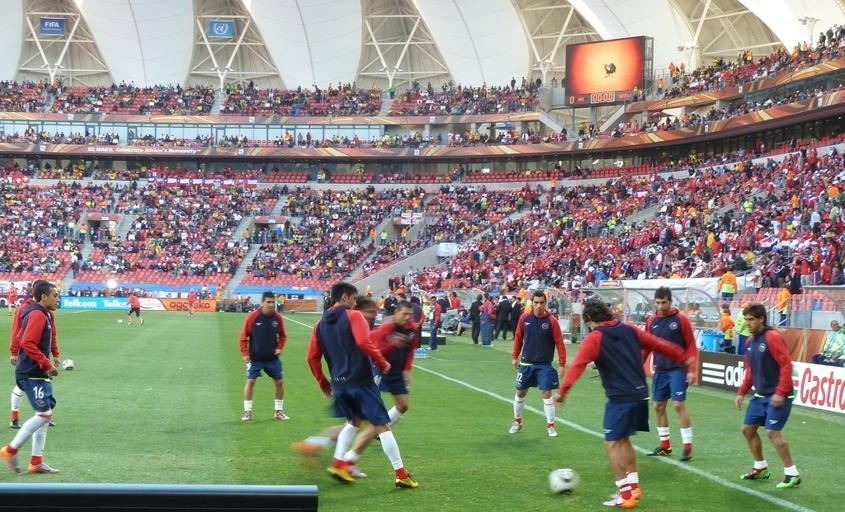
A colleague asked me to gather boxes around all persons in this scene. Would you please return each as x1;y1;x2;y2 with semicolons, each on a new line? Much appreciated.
9;287;59;428
308;282;419;488
371;301;419;441
509;292;567;437
0;282;60;475
555;300;695;510
293;296;407;478
735;303;803;489
642;287;696;461
0;23;844;364
240;291;290;421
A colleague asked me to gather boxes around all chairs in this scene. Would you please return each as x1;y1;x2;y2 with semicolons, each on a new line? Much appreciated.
225;87;383;118
0;75;215;118
610;115;664;136
2;119;601;150
759;125;844;161
7;147;763;184
664;44;845;128
0;154;845;311
384;78;558;118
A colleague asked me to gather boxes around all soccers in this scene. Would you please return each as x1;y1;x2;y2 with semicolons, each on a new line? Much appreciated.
61;358;74;371
548;467;577;498
118;318;123;323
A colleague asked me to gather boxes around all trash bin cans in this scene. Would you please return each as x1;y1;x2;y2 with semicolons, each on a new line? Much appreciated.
701;334;724;352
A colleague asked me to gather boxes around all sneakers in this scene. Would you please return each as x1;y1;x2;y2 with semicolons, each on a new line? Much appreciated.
0;418;60;474
775;474;801;488
548;426;557;436
326;462;418;489
740;468;771;480
241;409;252;420
647;446;672;456
274;410;289;420
509;421;522;433
602;488;641;508
